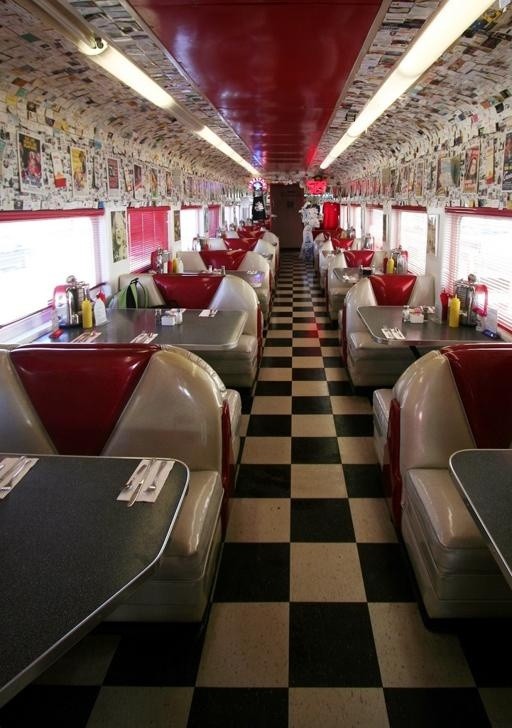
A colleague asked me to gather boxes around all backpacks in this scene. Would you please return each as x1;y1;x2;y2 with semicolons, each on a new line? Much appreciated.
108;278;148;309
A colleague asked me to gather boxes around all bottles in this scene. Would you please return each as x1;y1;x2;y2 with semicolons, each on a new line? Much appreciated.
359;251;409;275
162;250;226;275
81;288;183;329
402;289;461;328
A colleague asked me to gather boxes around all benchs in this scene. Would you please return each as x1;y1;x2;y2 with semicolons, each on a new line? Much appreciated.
310;226;512;635
0;220;279;624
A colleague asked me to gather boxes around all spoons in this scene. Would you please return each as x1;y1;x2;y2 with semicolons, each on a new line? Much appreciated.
121;464;148;492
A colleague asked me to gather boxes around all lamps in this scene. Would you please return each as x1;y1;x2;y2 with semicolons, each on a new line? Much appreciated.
10;1;497;180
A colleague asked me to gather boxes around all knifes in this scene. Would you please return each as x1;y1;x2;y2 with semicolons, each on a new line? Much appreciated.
127;457;158;508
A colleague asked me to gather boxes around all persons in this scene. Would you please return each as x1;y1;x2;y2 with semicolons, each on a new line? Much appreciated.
19;138;42;188
134;166;142;190
77;151;88;176
465;156;478;184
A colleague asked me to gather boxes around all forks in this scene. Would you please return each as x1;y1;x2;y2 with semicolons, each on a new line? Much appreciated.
0;459;31;499
144;460;168;496
